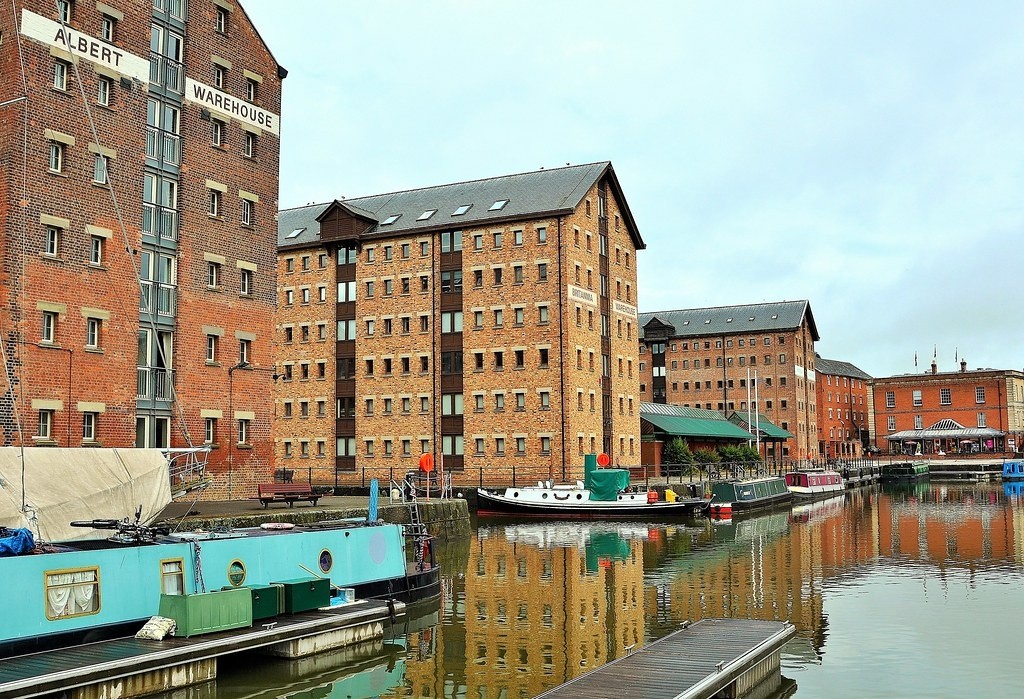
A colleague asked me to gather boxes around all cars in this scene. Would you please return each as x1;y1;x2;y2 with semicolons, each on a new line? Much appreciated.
864;446;882;457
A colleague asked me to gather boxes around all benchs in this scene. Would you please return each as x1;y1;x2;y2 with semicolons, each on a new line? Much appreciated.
409;470;438;485
274;470;294;483
705;465;722;478
249;483;322;510
755;463;768;475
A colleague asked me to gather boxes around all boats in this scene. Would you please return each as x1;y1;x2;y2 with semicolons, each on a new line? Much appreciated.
888;462;930;477
475;467;713;519
784;468;846;494
0;493;444;699
1001;460;1024;482
683;475;793;514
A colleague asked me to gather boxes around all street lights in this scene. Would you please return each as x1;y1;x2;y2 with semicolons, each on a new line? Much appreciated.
226;360;250;503
860;422;865;462
